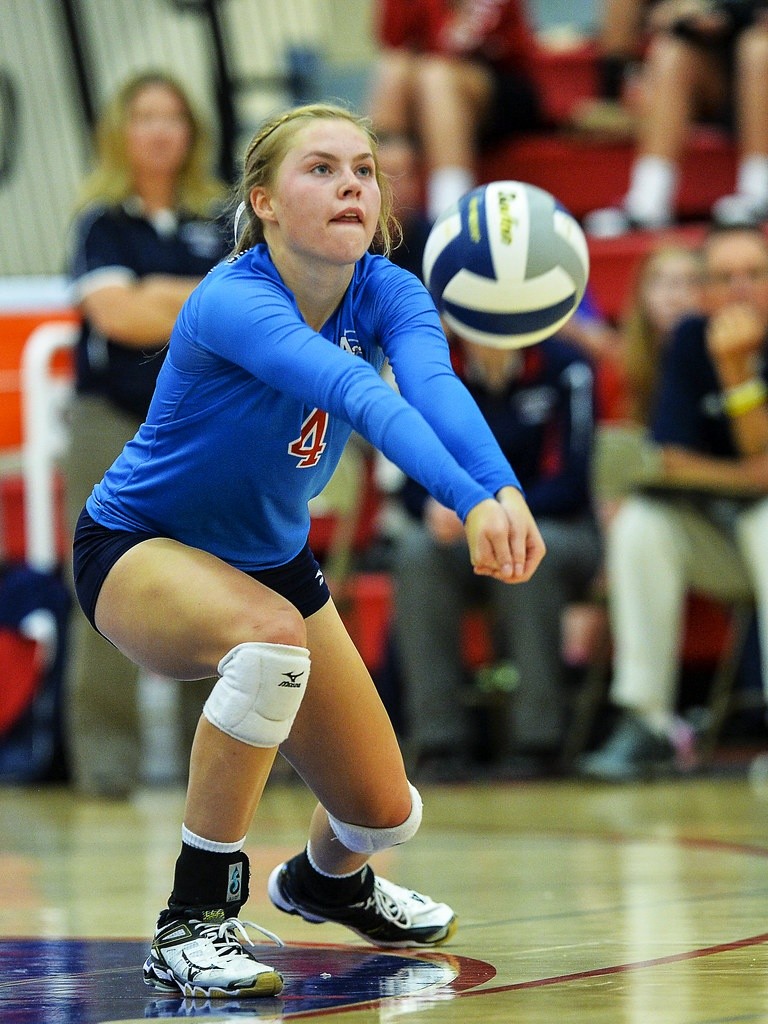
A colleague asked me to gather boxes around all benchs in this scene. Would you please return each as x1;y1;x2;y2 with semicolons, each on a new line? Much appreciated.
306;112;768;671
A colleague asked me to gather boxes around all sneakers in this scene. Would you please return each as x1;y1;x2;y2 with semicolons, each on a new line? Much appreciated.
264;859;458;946
293;949;462;1009
143;994;285;1023
139;909;285;1000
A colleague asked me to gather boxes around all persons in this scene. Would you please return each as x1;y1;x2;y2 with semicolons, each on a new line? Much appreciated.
1;0;767;801
73;105;552;999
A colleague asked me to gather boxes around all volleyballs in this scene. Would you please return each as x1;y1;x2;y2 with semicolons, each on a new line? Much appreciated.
420;180;591;352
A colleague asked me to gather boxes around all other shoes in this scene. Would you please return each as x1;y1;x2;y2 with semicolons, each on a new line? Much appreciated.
574;712;705;784
581;201;646;238
70;766;132;798
403;737;471;780
498;736;559;781
710;189;764;225
